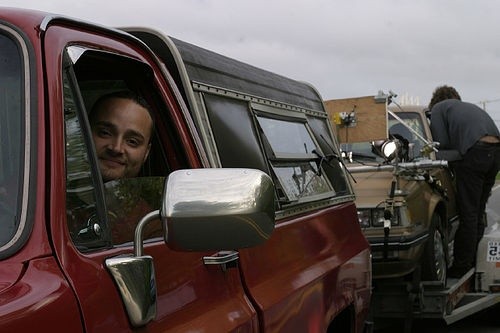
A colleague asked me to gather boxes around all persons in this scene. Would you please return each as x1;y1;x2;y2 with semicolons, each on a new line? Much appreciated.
390;123;424;161
428;84;500;277
0;90;162;253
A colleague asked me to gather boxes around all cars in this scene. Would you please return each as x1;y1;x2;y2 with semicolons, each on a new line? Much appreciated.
0;5;371;333
332;103;463;290
